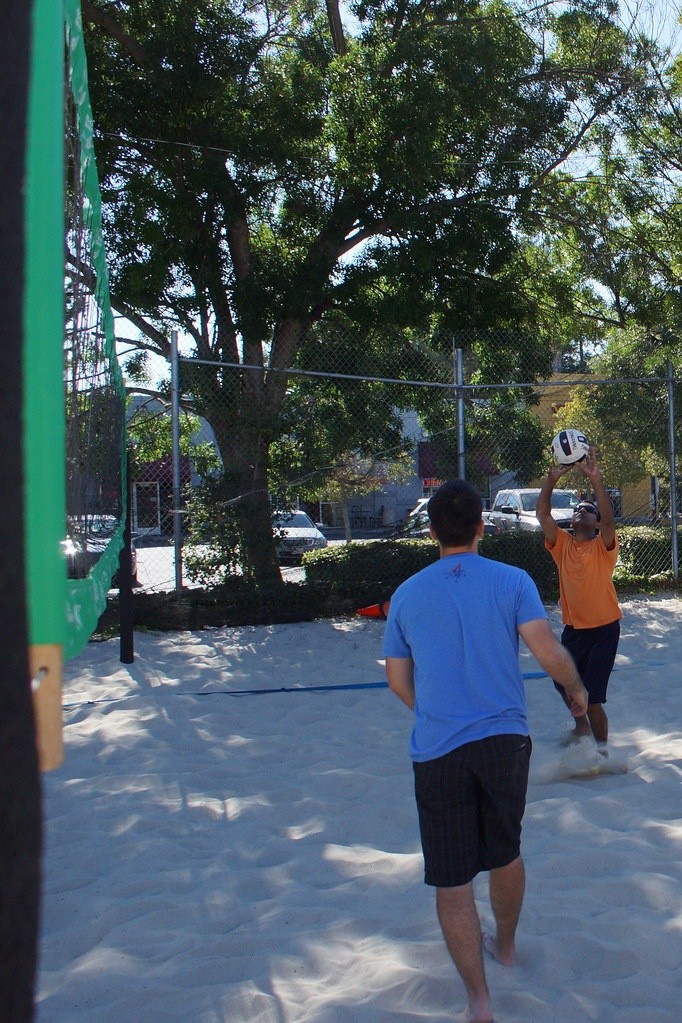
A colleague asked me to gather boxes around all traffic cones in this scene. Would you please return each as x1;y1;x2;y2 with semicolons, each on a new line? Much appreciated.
357;598;390;620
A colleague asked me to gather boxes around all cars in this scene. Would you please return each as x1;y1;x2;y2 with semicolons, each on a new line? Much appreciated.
271;511;329;565
66;514;140;587
406;499;500;542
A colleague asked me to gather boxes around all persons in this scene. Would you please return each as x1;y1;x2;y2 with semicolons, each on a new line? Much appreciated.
380;483;589;1023
536;447;623;759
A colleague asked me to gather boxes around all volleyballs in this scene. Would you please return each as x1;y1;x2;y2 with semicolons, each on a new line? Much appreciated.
550;429;590;467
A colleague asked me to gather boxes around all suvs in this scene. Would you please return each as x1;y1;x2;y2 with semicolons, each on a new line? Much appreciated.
489;489;582;538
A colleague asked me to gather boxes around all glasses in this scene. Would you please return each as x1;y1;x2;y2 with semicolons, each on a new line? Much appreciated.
575;506;598;520
